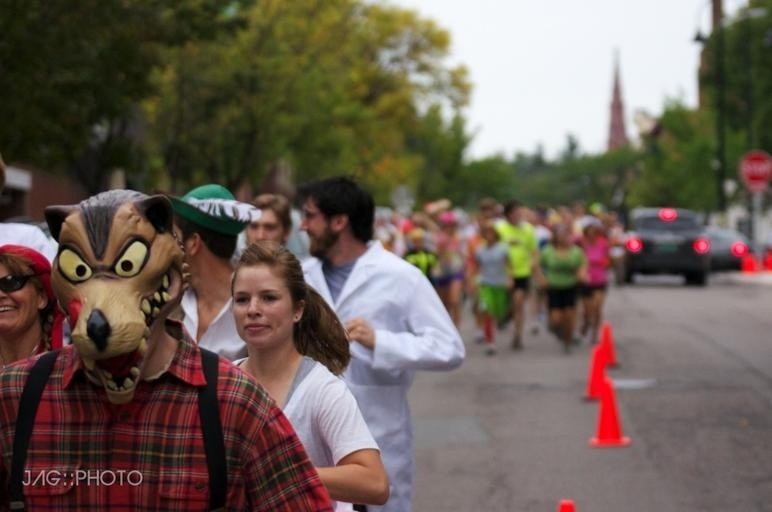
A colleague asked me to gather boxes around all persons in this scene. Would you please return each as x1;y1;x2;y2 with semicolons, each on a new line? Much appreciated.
295;172;466;512
375;195;629;352
228;238;391;512
245;192;293;250
168;182;260;364
1;187;336;512
0;223;61;363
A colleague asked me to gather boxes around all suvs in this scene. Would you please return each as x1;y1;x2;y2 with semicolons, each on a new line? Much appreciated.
622;206;707;286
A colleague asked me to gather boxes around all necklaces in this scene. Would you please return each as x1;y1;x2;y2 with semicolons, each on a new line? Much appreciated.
0;330;43;372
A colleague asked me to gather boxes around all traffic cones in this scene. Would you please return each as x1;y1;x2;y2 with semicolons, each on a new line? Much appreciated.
591;318;618;368
743;247;772;272
580;341;618;402
584;378;632;448
555;498;578;511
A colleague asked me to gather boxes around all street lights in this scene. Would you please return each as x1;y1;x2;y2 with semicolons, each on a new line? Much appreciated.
693;0;753;237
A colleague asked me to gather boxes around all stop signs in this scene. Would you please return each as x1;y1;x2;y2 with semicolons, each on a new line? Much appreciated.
742;151;771;185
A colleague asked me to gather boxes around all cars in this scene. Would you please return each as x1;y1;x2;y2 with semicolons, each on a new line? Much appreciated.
705;229;753;271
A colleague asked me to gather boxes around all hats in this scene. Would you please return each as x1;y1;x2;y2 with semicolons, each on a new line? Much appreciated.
166;183;249;237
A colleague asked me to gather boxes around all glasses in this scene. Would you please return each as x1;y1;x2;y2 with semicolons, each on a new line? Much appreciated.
0;272;40;294
